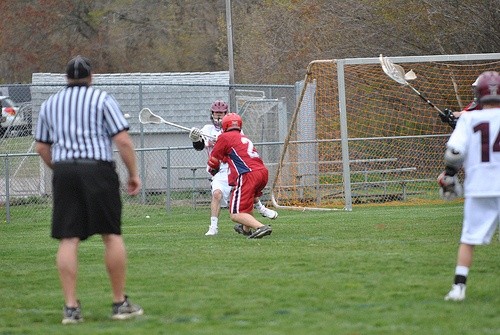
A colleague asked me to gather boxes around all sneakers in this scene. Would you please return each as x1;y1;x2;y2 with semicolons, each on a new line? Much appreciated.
111;293;143;320
233;224;253;236
247;225;273;239
258;205;278;220
444;282;466;303
204;225;217;236
63;300;84;325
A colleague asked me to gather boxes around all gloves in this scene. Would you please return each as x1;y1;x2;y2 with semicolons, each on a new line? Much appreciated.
435;165;462;200
188;127;203;142
439;108;455;122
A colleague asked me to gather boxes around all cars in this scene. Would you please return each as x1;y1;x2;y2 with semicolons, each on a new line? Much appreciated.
0;96;24;126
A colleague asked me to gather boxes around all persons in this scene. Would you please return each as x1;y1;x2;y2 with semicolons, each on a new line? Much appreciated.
439;92;479;124
207;112;272;240
189;99;278;236
437;70;500;301
33;55;143;324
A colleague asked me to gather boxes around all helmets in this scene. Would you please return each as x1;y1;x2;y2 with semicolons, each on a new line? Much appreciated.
475;71;500;103
210;100;229;128
221;112;242;132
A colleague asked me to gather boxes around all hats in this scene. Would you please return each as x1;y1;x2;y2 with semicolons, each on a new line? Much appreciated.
66;55;91;78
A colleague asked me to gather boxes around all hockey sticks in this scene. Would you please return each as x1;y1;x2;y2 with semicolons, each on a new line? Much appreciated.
138;108;217;140
380;55;451;120
